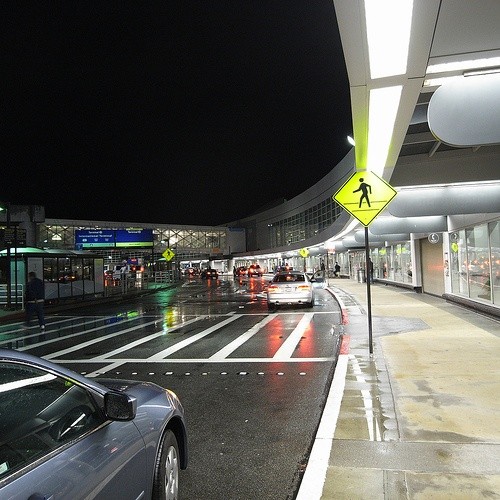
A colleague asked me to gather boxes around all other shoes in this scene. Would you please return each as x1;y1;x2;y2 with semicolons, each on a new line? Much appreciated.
23;323;32;327
40;325;45;329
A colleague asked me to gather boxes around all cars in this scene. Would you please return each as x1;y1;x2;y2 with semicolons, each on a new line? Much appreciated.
0;349;189;500
247;265;263;277
236;266;248;277
179;266;218;280
267;270;325;310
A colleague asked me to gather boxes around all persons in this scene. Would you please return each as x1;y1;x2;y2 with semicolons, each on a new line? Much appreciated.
333;262;340;278
232;265;237;279
321;263;326;271
208;265;212;270
22;271;46;330
286;263;288;266
